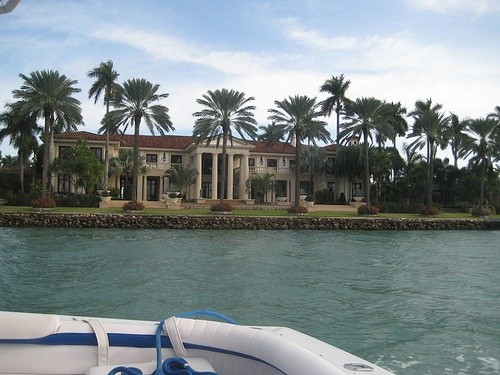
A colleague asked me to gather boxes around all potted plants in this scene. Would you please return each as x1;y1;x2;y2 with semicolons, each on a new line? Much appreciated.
299;188;307;200
277;193;287;202
101;191;110;202
353;194;363;201
168;192;178;204
